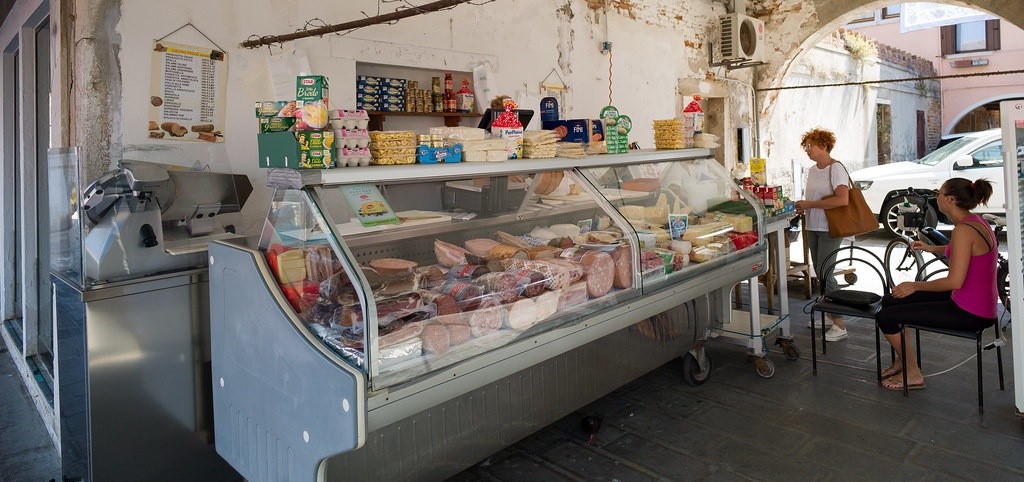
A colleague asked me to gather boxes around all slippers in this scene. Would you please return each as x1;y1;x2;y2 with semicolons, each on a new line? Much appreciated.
881;367;902;378
881;376;927;390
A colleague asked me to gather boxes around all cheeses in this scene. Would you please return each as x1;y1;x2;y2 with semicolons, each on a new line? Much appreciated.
620;204;753;265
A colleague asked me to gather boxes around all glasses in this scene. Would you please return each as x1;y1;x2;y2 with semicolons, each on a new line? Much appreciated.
803;143;825;151
933;189;945;196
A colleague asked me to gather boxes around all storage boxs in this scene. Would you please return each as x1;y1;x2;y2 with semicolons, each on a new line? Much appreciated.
357;75;434;113
255;75;336;168
541;119;608;155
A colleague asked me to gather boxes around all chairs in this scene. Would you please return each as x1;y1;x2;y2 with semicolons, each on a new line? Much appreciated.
900;256;1005;414
803;244;896;386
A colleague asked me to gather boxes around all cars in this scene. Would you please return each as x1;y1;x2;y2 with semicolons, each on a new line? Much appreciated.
847;129;1007;244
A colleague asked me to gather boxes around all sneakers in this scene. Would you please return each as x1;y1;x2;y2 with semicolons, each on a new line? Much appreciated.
808;316;834;328
821;325;848;341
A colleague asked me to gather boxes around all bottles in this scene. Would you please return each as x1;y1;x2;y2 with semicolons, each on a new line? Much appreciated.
742;176;754;194
431;76;443;112
456;79;475;114
442;73;457;113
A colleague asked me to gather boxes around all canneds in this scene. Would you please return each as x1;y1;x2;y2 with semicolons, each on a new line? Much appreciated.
404;80;436;112
431;73;456;113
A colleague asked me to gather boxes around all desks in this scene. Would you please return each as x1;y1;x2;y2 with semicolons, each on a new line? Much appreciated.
735;209;811;314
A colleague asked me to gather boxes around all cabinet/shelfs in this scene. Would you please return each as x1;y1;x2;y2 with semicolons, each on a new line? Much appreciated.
708;215;799;378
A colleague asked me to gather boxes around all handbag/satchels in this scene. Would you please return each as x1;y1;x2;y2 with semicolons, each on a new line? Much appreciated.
821;161;880;239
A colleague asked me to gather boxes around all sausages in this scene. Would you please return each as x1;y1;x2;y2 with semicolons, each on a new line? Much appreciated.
150;96;215;135
417;247;630;356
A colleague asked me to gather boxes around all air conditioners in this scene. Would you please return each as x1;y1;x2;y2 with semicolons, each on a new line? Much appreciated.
718;13;767;68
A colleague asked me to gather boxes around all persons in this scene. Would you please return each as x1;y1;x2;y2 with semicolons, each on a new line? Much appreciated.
794;129;850;343
876;177;999;388
